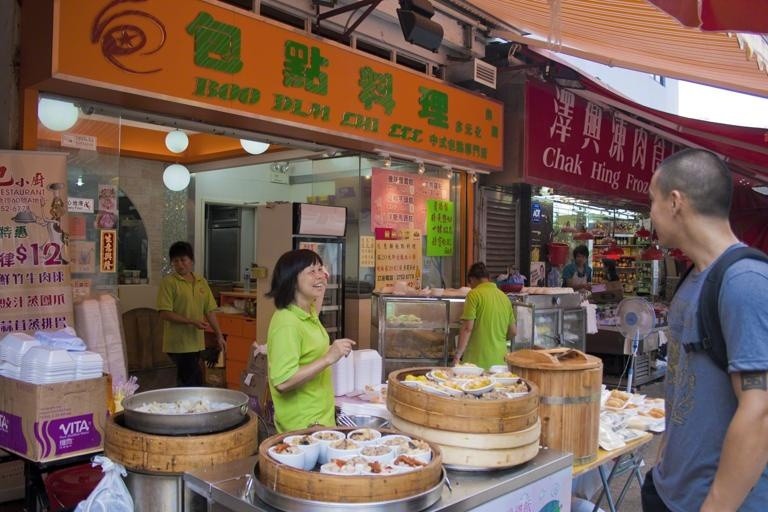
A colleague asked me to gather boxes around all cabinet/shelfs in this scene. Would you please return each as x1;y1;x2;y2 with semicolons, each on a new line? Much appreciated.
512;302;588;357
372;292;516;382
198;288;258;390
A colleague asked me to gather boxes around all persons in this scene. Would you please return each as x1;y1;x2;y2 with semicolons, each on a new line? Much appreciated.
263;248;358;435
155;240;227;386
639;148;767;511
450;262;517;372
602;258;621;282
562;244;594;292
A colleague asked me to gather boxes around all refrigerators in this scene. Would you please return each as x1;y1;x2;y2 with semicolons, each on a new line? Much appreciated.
253;202;348;355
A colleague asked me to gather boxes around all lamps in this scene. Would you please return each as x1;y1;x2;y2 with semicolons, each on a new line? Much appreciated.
312;0;447;52
483;39;586;90
375;147;491;185
165;125;189;155
553;199;690;262
160;151;191;191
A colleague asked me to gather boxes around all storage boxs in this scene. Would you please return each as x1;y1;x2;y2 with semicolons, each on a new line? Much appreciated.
238;337;279;436
0;371;108;464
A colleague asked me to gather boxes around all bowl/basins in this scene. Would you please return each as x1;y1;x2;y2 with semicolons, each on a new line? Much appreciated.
121;386;252;433
495;282;523;293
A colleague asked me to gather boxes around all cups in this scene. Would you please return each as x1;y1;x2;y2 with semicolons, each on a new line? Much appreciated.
268;429;431;476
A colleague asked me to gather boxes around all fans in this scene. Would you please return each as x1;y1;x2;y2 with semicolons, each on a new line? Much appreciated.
614;293;657;394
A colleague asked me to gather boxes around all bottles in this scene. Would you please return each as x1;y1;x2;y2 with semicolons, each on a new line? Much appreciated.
592;234;652;293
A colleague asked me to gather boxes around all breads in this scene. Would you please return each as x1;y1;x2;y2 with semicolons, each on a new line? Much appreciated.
605;389;664;418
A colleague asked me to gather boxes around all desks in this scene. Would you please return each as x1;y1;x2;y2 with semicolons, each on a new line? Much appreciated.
598;386;666;489
572;428;658;512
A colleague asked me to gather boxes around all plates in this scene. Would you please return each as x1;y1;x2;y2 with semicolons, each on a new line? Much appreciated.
402;365;530;401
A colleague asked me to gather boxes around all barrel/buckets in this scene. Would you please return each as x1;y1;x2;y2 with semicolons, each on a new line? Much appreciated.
503;347;604;468
